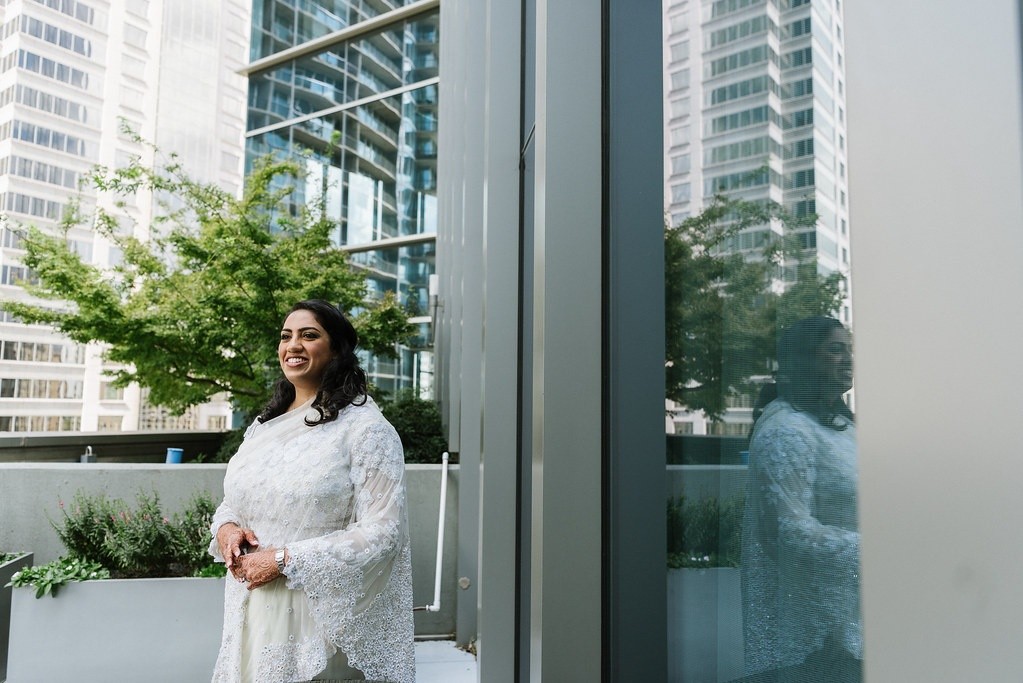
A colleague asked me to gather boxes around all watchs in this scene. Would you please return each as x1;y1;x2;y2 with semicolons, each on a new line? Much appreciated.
273;546;285;575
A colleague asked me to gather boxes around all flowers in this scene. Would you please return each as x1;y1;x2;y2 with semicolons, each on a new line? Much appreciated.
3;490;248;598
666;491;747;567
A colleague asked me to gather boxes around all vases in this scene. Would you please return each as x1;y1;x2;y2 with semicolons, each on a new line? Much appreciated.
670;566;744;682
3;576;228;683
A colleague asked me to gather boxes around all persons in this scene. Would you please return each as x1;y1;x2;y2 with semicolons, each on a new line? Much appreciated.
205;297;417;683
737;314;862;683
